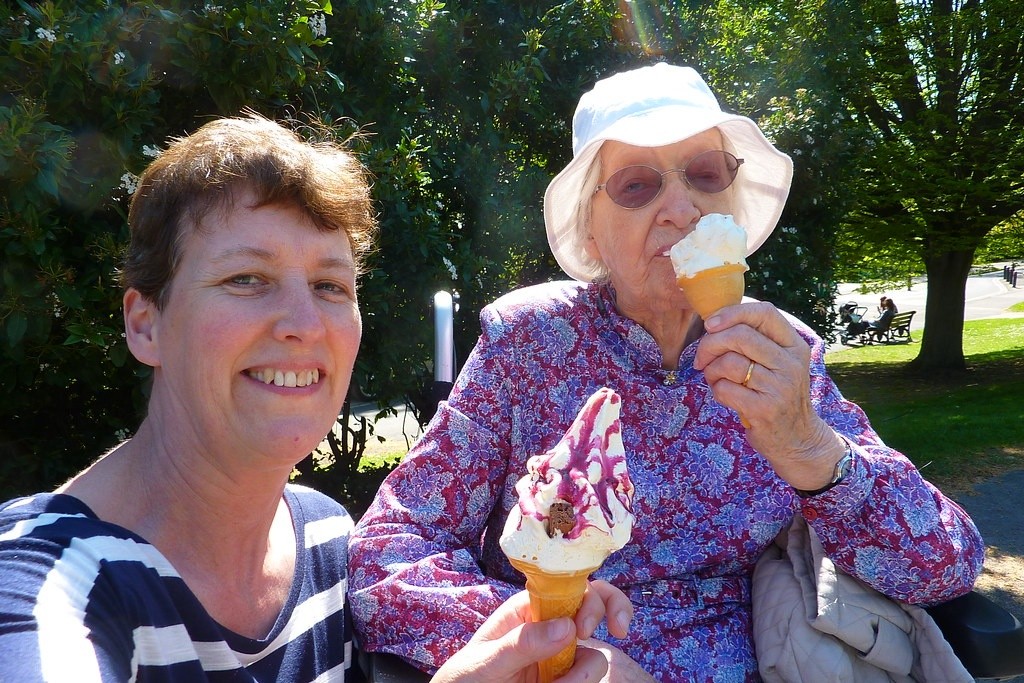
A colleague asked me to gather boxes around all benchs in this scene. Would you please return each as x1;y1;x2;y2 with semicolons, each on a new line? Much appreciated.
867;310;917;345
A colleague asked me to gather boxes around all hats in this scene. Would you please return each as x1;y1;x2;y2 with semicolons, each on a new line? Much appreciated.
543;62;794;287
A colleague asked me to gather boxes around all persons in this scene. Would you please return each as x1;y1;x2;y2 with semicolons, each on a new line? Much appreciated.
0;106;635;683
872;295;899;340
349;61;986;683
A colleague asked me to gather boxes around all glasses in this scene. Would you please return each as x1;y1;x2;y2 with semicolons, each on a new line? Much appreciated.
591;150;744;209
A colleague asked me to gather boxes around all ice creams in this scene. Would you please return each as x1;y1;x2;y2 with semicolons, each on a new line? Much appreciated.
500;387;636;682
667;213;746;322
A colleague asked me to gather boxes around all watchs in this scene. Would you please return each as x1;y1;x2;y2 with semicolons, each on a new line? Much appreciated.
790;445;852;498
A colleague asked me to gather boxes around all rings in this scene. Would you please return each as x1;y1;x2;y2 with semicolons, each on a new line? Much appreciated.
741;360;755;387
